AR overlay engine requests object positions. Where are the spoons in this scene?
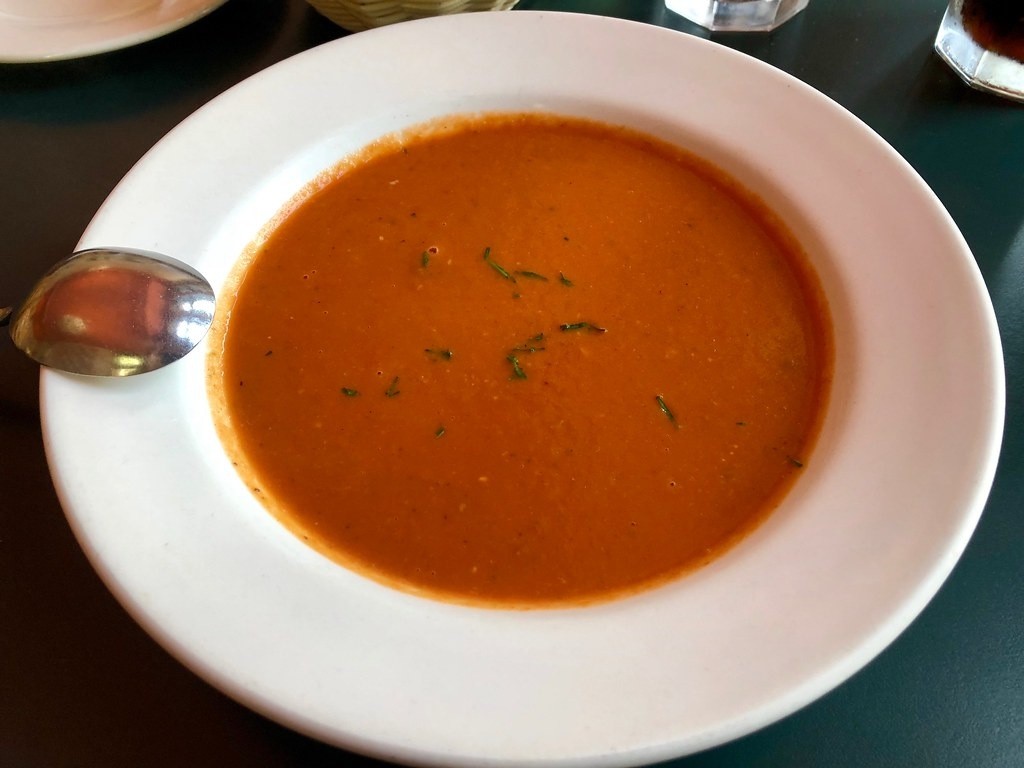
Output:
[0,245,217,378]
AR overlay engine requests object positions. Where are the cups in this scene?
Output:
[932,0,1024,99]
[663,0,809,32]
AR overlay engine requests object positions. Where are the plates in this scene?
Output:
[38,10,1006,768]
[0,0,228,64]
